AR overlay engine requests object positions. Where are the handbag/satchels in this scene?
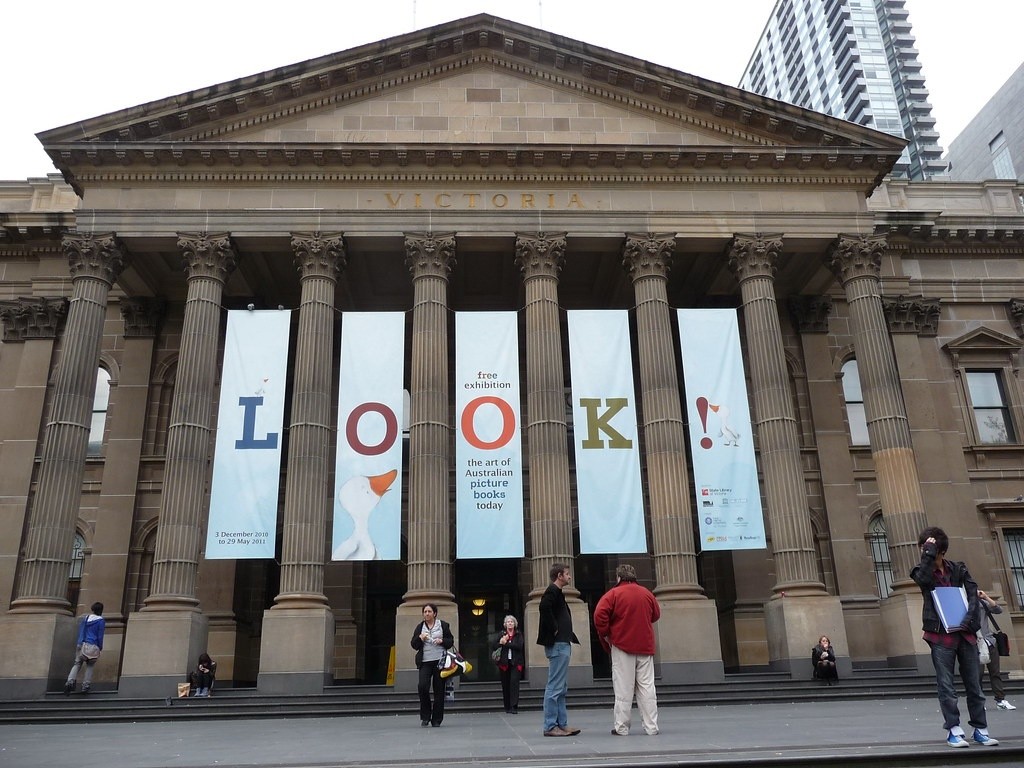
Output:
[438,644,473,678]
[994,631,1010,656]
[977,635,994,664]
[491,630,504,661]
[80,642,100,664]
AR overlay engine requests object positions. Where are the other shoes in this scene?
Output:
[432,722,440,727]
[420,720,430,726]
[824,679,835,686]
[63,683,73,697]
[611,729,619,735]
[543,726,581,736]
[506,708,518,714]
[194,687,208,697]
[81,684,92,692]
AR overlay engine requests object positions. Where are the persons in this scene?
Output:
[536,563,581,736]
[594,564,660,735]
[492,615,523,714]
[63,602,105,697]
[910,526,999,748]
[193,653,217,696]
[976,590,1016,711]
[410,603,454,728]
[812,636,839,686]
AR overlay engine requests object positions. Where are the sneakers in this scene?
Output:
[996,700,1016,710]
[946,726,969,748]
[970,727,999,746]
